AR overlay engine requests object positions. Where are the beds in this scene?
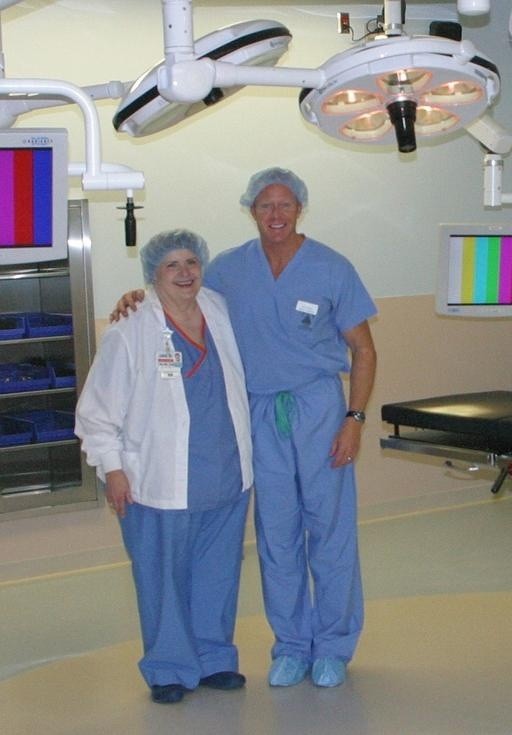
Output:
[380,388,511,494]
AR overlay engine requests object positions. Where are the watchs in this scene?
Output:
[346,410,367,421]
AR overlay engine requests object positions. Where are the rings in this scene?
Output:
[344,455,353,462]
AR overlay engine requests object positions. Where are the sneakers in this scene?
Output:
[150,685,184,705]
[198,672,246,691]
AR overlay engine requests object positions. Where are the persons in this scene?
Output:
[74,229,255,704]
[106,166,379,690]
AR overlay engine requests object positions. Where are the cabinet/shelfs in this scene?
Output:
[0,200,96,517]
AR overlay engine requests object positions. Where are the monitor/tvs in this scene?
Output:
[435,223,512,318]
[0,128,69,281]
